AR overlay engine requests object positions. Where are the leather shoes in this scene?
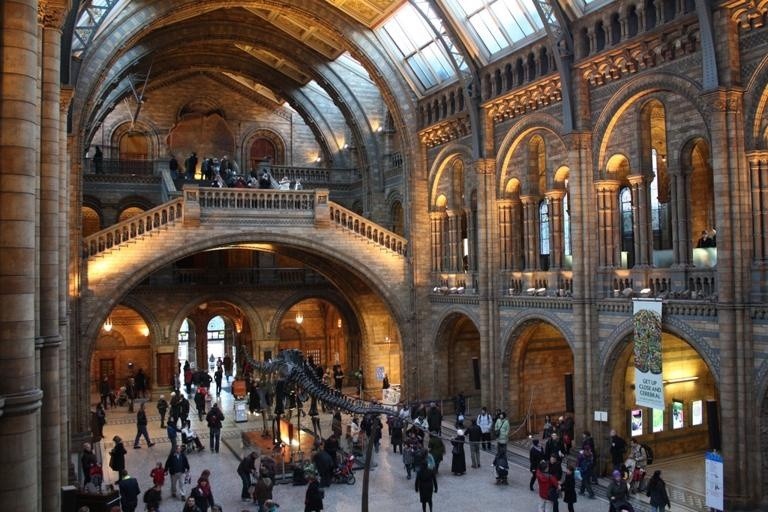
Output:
[148,443,155,447]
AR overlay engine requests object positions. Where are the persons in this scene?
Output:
[708,226,715,247]
[386,391,510,485]
[415,461,439,511]
[157,351,391,511]
[168,152,272,189]
[695,228,712,249]
[92,145,105,176]
[73,367,167,511]
[528,414,671,511]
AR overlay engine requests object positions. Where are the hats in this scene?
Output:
[160,394,164,399]
[170,391,176,396]
[613,470,620,477]
[610,429,616,436]
[133,446,141,449]
[456,429,464,436]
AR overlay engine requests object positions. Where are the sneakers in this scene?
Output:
[172,493,176,496]
[181,495,185,501]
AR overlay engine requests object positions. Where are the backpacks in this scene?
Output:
[640,442,653,465]
[478,413,490,425]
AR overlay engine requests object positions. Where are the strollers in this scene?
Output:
[333,454,356,485]
[623,456,638,496]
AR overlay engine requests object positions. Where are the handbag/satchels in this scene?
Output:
[494,429,500,436]
[547,485,558,499]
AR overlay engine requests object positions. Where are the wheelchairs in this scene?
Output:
[179,427,203,453]
